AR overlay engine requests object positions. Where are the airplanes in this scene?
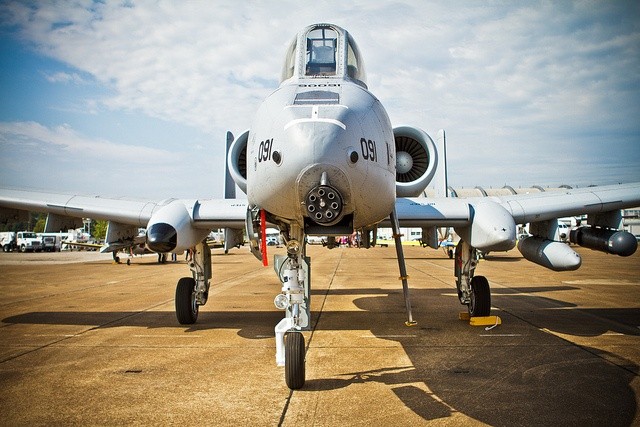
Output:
[370,227,519,258]
[0,25,640,389]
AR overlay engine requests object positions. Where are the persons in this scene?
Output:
[306,64,312,76]
[347,65,368,89]
[171,253,178,262]
[184,249,195,261]
[158,252,167,263]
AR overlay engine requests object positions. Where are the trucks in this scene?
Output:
[0,230,44,252]
